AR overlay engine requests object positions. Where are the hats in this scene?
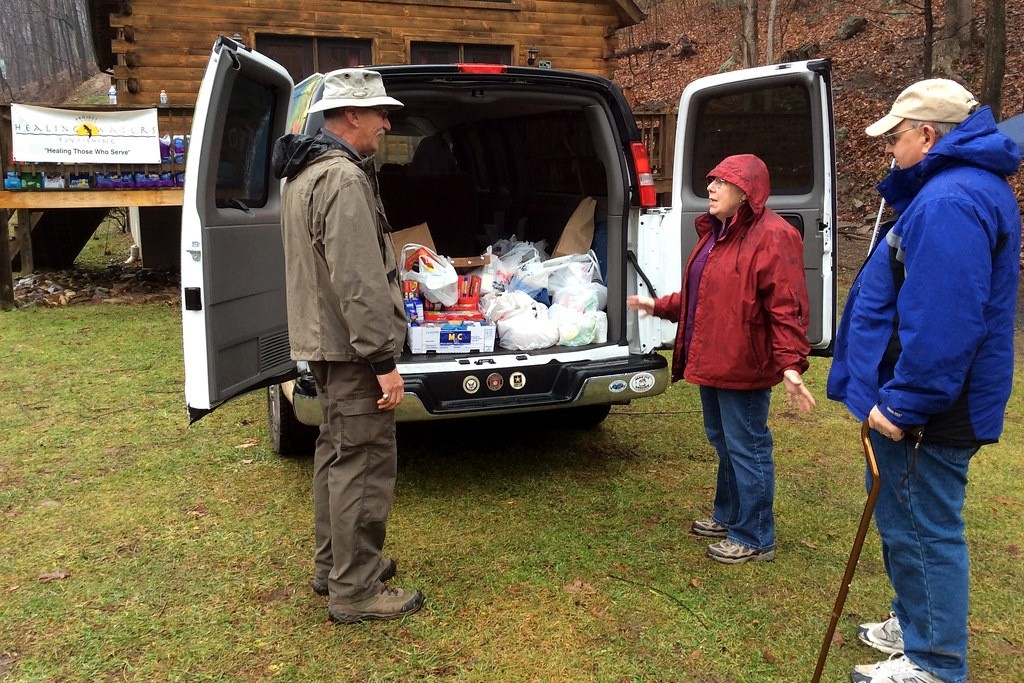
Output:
[865,78,979,138]
[305,68,405,114]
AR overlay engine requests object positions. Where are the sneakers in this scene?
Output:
[851,654,947,683]
[329,584,424,625]
[312,557,397,597]
[691,518,730,538]
[857,611,906,657]
[706,538,776,564]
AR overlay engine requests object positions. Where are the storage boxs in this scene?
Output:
[406,304,499,355]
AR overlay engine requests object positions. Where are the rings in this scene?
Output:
[380,393,393,401]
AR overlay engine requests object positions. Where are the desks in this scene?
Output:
[0,188,185,308]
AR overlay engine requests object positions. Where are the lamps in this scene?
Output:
[527,43,538,65]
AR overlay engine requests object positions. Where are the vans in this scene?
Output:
[179,33,839,457]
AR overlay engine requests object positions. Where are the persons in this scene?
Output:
[273,67,429,626]
[630,154,817,566]
[828,76,1024,683]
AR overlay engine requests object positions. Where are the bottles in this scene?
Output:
[160,90,167,104]
[108,86,117,104]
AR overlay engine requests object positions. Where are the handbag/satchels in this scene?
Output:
[479,242,608,351]
[550,196,597,258]
[399,244,459,308]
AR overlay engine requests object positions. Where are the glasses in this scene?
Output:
[882,125,915,146]
[706,176,728,191]
[363,107,388,118]
